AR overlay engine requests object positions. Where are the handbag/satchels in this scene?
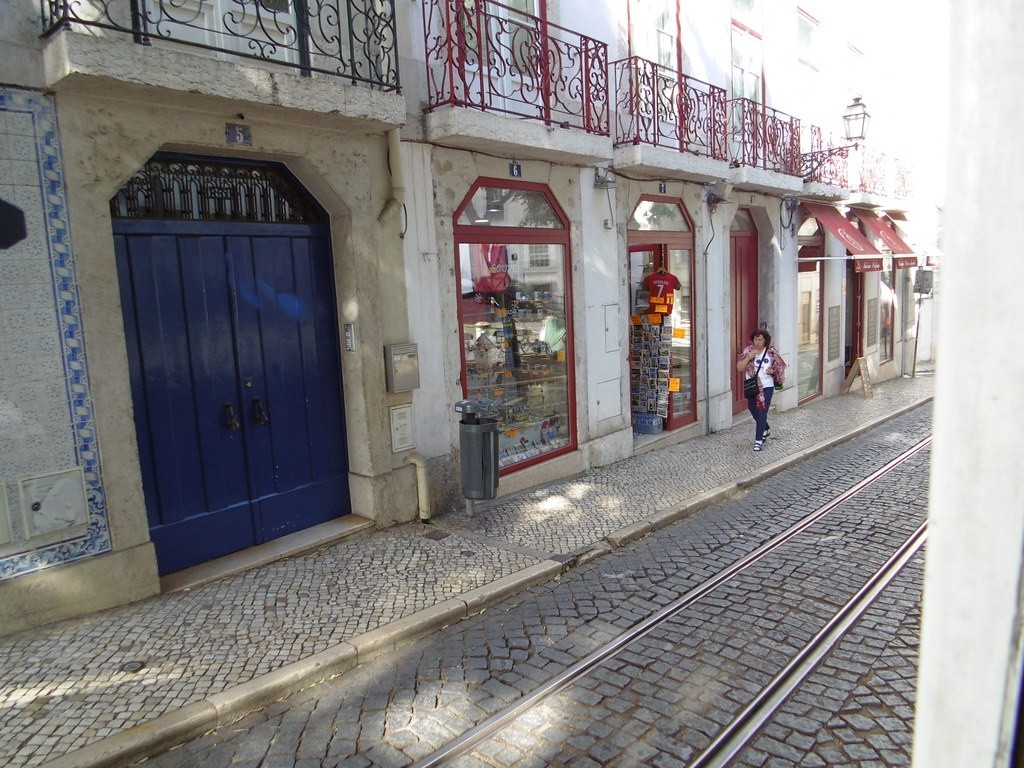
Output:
[744,377,760,398]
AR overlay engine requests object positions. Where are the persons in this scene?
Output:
[737,328,786,451]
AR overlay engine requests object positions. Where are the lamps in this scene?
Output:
[707,181,734,209]
[795,97,871,177]
[593,167,617,191]
[782,195,799,211]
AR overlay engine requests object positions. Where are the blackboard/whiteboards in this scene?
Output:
[842,357,872,394]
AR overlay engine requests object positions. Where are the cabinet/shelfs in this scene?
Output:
[468,375,568,464]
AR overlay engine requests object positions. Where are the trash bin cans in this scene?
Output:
[454,398,499,501]
[474,321,491,339]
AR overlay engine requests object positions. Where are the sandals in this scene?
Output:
[761,429,770,443]
[754,442,762,451]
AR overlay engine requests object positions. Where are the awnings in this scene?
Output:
[889,208,942,267]
[850,201,918,271]
[798,199,885,275]
